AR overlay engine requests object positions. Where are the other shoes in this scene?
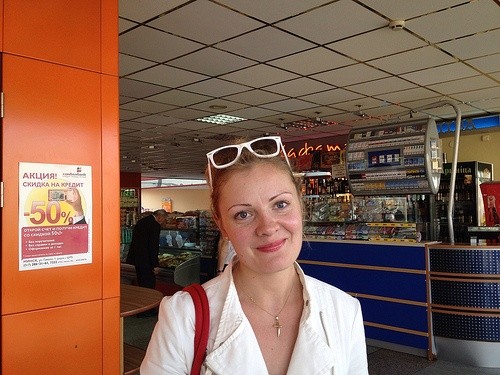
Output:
[137,309,156,318]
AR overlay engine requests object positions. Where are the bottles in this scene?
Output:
[435,182,472,202]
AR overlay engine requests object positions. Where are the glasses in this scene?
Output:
[206,136,294,190]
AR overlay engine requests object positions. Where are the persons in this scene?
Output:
[124,209,169,292]
[140,135,368,375]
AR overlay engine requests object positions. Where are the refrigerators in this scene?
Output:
[437,161,494,243]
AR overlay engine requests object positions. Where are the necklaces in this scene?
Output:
[232,275,298,336]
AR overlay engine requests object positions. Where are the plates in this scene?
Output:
[478,243,487,246]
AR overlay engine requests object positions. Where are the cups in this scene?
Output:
[479,239,486,243]
[470,236,477,246]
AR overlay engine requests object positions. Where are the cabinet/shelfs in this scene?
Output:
[120,282,163,375]
[118,174,427,290]
